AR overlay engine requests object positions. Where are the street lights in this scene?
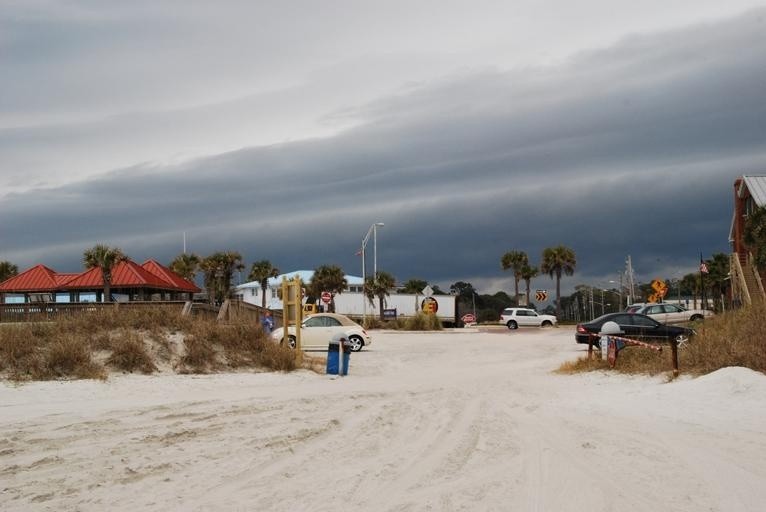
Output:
[373,222,386,281]
[581,279,638,315]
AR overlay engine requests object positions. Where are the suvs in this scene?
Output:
[498,306,560,330]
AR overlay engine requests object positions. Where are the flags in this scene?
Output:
[700,260,711,275]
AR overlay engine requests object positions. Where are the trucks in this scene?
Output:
[301,292,460,330]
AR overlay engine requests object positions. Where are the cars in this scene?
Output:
[632,303,715,326]
[267,314,372,354]
[574,313,698,350]
[622,301,644,314]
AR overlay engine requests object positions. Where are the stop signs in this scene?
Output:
[319,291,333,304]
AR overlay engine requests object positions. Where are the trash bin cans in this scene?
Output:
[598,321,625,360]
[326,333,351,376]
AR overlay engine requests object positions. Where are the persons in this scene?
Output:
[260,309,275,333]
